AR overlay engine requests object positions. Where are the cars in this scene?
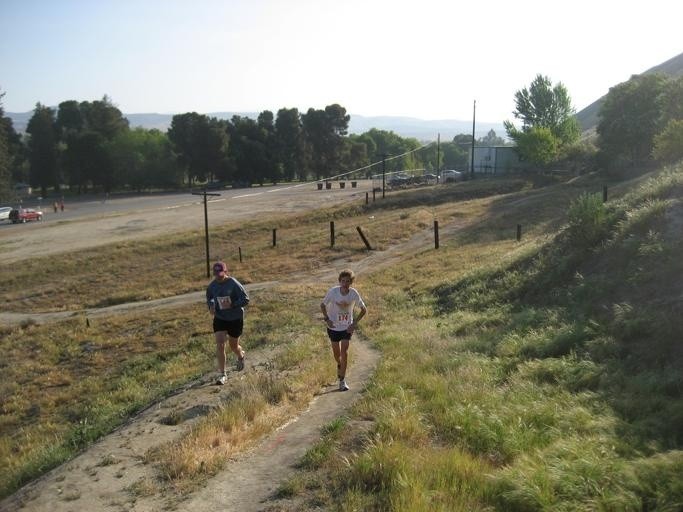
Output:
[388,169,462,186]
[0,205,43,225]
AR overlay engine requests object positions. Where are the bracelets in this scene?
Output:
[324,316,329,321]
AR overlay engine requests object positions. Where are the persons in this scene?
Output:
[54,200,58,212]
[59,198,64,212]
[207,263,249,385]
[321,271,366,391]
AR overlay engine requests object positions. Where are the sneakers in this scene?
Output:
[337,362,342,378]
[236,350,246,371]
[339,378,349,391]
[216,370,228,385]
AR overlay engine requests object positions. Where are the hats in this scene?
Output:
[213,262,227,276]
